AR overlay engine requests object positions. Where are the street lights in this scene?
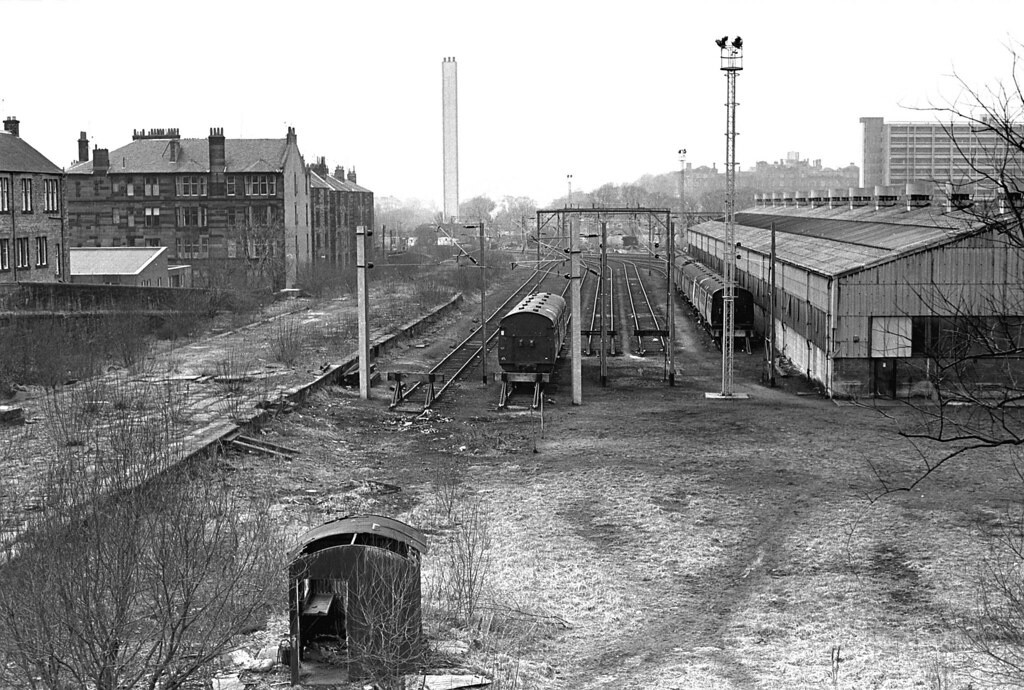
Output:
[678,146,687,241]
[716,35,742,398]
[566,171,575,212]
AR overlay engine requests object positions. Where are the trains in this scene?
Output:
[667,248,756,344]
[491,291,569,385]
